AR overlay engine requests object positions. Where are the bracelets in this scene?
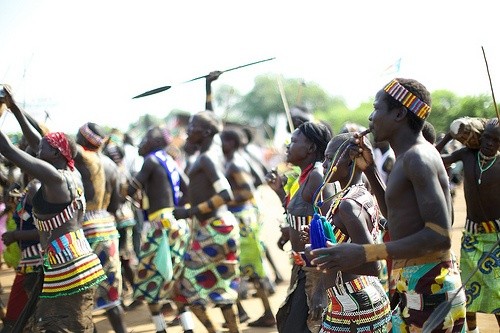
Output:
[227,200,238,206]
[187,209,193,218]
[281,194,289,208]
[383,220,388,232]
[362,242,388,264]
[206,94,212,103]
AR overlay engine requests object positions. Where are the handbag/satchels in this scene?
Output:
[275,268,312,333]
[3,272,29,321]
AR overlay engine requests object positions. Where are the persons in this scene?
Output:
[309,77,466,333]
[422,114,500,333]
[0,70,396,333]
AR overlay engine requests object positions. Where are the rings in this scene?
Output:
[268,178,272,182]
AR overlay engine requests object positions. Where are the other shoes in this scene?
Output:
[247,315,276,327]
[166,316,180,326]
[240,313,249,323]
[252,286,276,297]
[123,299,143,311]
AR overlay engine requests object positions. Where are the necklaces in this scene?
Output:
[343,182,365,192]
[477,150,499,185]
